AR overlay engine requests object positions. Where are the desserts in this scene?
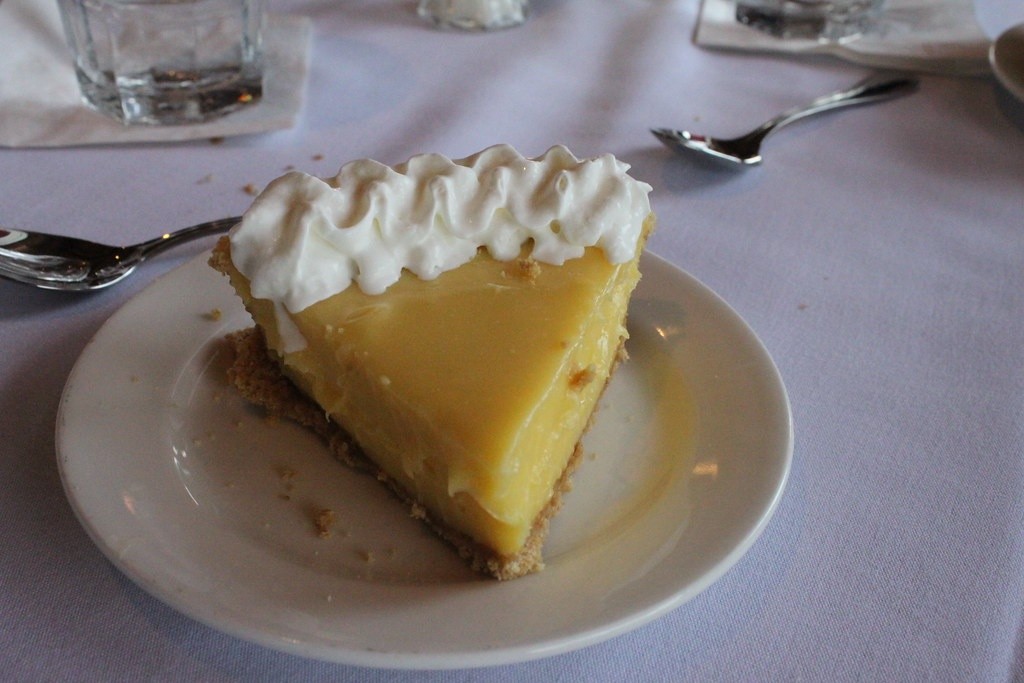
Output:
[207,144,656,583]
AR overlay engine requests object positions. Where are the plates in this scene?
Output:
[54,247,795,671]
[987,21,1024,105]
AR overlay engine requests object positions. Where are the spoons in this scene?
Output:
[0,216,243,290]
[650,69,921,170]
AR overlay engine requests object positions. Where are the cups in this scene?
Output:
[734,0,887,45]
[55,0,265,126]
